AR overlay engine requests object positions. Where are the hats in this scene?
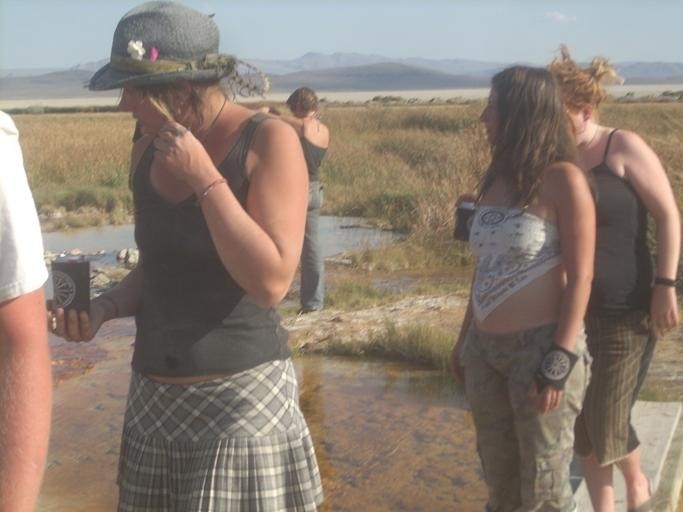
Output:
[86,0,238,91]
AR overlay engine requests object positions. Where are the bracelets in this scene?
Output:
[195,179,227,207]
[655,277,680,288]
[102,295,120,317]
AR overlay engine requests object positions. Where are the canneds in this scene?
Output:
[50,251,89,318]
[454,202,474,241]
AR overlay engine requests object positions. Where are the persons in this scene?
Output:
[458,46,682,512]
[259,86,330,313]
[1,111,52,512]
[450,64,596,512]
[45,1,325,510]
